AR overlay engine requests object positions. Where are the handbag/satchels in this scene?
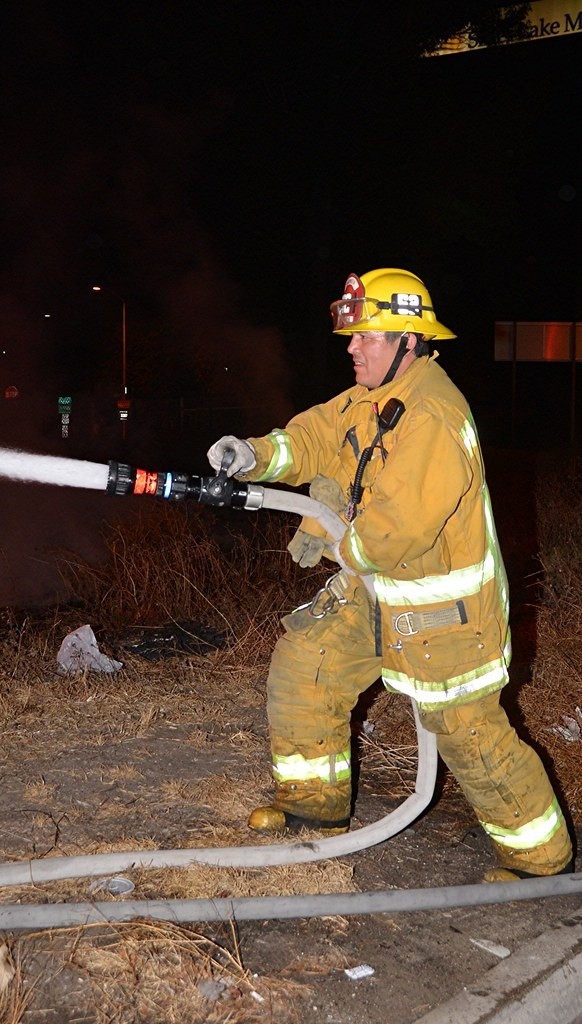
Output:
[57,624,123,677]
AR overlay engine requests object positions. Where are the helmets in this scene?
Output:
[330,267,457,342]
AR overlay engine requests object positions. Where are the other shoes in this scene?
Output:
[482,865,574,884]
[247,805,350,835]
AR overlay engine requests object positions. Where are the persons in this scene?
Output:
[205,267,576,884]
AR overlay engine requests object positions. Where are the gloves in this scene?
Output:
[206,435,257,478]
[287,473,346,568]
